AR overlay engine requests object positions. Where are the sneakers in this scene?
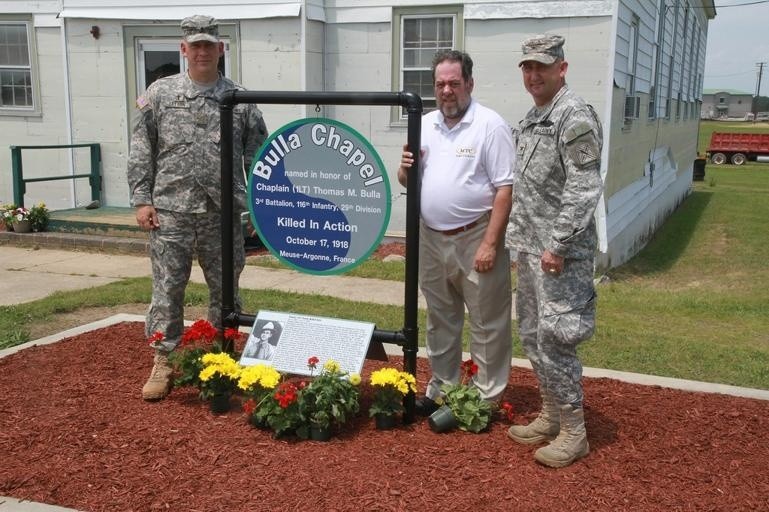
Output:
[415,395,438,415]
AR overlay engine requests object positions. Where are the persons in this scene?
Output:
[506,32,604,469]
[244,321,274,360]
[127,14,269,400]
[396,50,515,417]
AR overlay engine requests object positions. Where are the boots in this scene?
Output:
[508,398,560,444]
[143,350,175,400]
[534,399,589,468]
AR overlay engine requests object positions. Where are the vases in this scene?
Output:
[7,222,44,232]
[3,203,50,229]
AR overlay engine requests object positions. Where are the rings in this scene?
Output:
[550,269,555,272]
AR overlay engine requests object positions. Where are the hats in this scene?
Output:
[260,322,278,334]
[519,33,564,67]
[181,14,219,43]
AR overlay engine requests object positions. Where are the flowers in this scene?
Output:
[147,319,250,353]
[199,352,281,391]
[370,366,418,396]
[275,382,298,410]
[496,400,513,422]
[243,398,259,417]
[300,355,362,393]
[458,357,480,386]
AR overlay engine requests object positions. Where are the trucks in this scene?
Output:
[706,132,769,166]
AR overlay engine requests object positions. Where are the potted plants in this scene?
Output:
[427,403,456,434]
[374,409,398,432]
[206,390,230,418]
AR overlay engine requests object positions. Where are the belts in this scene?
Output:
[427,221,476,236]
